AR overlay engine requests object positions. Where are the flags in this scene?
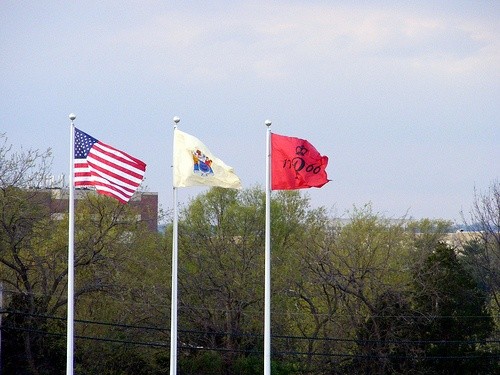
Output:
[269,132,331,190]
[173,127,243,190]
[73,126,147,206]
